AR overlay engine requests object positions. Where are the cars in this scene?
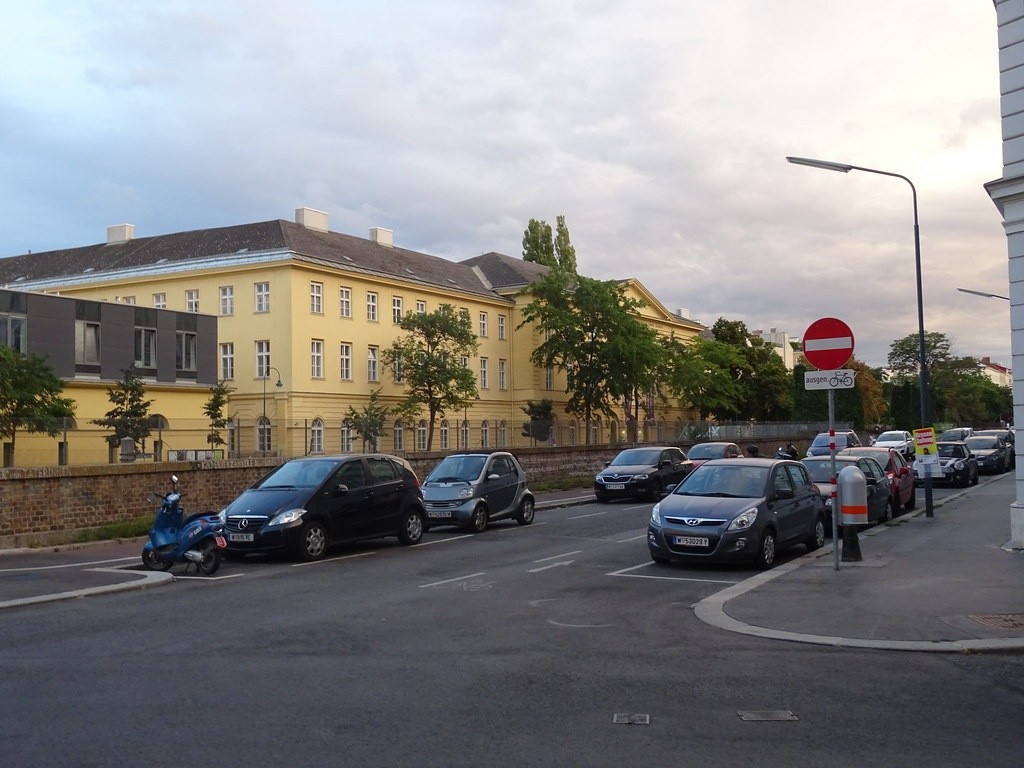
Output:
[593,446,697,504]
[835,445,917,518]
[419,450,537,534]
[940,426,1015,468]
[871,429,915,462]
[646,456,828,571]
[788,455,897,540]
[962,434,1010,474]
[687,442,745,468]
[911,440,978,489]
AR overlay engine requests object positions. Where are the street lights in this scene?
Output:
[262,366,285,456]
[786,154,934,518]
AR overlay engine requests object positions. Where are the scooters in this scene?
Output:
[140,475,229,576]
[747,441,800,462]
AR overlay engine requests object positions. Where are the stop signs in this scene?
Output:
[800,317,855,371]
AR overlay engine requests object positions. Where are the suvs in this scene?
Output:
[215,453,427,562]
[805,429,863,457]
